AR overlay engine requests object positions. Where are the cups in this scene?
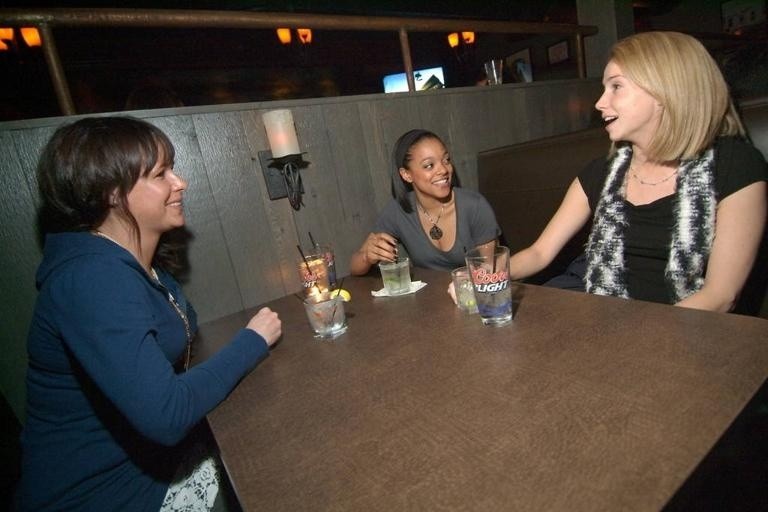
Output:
[485,59,502,85]
[298,245,350,341]
[452,243,512,329]
[379,257,414,295]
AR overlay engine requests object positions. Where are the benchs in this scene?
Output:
[476,104,766,314]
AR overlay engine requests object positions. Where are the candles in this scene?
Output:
[260,108,302,160]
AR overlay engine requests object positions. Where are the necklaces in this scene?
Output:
[626,162,679,185]
[419,204,446,240]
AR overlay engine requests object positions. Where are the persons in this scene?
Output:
[20,115,285,512]
[445,30,767,314]
[349,130,516,273]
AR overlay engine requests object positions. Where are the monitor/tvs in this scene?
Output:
[379,61,449,93]
